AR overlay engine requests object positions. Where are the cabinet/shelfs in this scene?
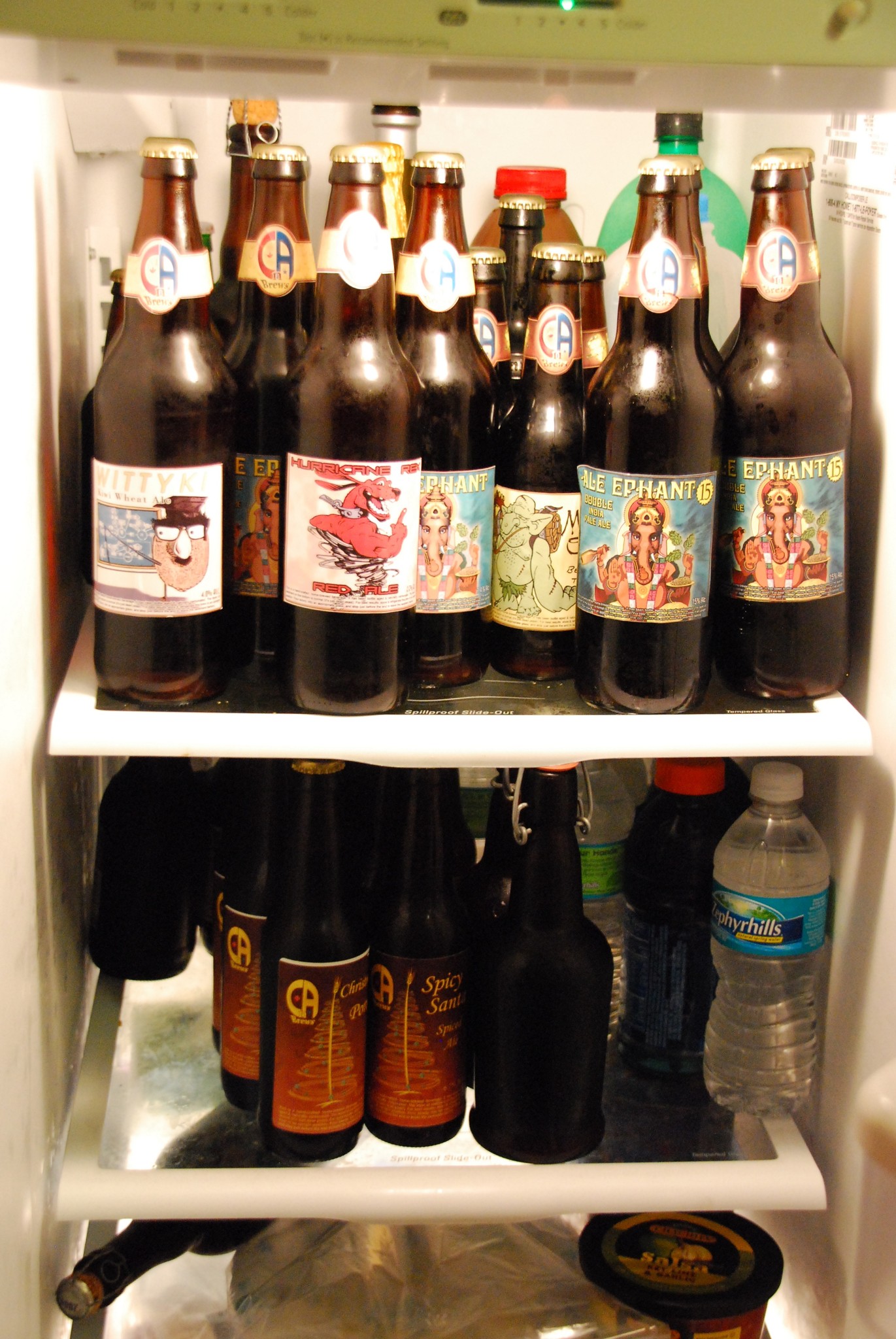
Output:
[36,579,877,1213]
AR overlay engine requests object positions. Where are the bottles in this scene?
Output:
[80,138,226,707]
[371,103,421,224]
[208,98,282,339]
[574,759,653,1042]
[483,242,609,682]
[280,142,428,716]
[717,148,853,701]
[198,757,257,1113]
[614,757,732,1087]
[396,152,497,692]
[221,144,316,662]
[256,757,365,1163]
[576,155,726,715]
[469,166,584,369]
[85,757,198,980]
[702,762,832,1118]
[55,1100,280,1322]
[469,245,512,434]
[364,764,475,1148]
[469,765,614,1164]
[598,113,750,354]
[485,195,546,434]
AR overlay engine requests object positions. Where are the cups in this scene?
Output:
[578,1212,785,1339]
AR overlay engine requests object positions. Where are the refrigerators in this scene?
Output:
[0,0,896,1338]
